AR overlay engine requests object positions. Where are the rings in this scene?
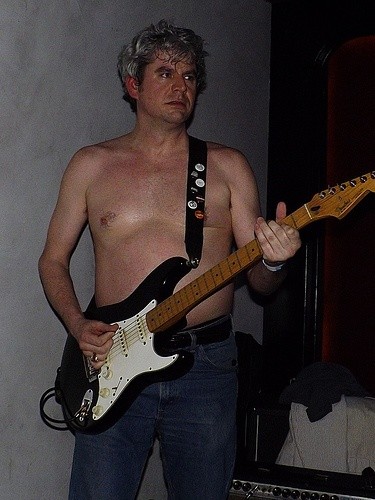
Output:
[91,352,98,362]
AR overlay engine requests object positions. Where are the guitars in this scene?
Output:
[55,171,375,435]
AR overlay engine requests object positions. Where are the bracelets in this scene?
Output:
[262,260,286,272]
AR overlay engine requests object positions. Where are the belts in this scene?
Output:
[170,316,231,348]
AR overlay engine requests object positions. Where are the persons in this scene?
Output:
[38,18,301,500]
